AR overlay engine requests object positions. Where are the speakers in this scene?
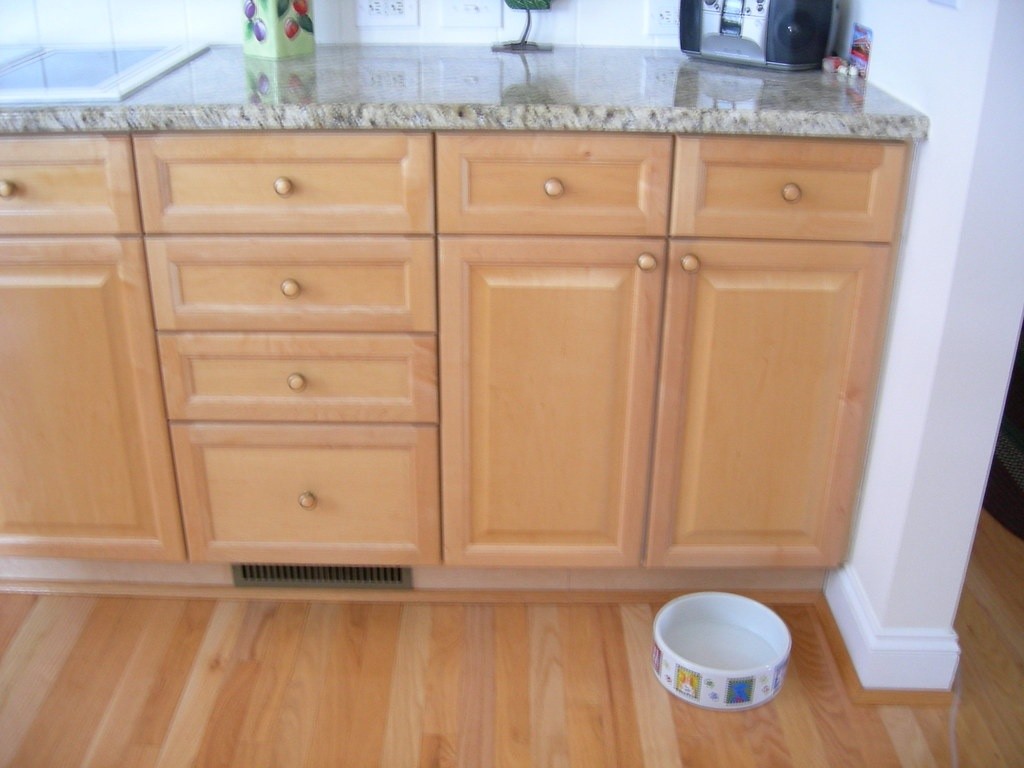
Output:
[767,0,840,70]
[679,0,702,59]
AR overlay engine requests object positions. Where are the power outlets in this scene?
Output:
[644,0,680,35]
[354,1,422,26]
[440,1,503,30]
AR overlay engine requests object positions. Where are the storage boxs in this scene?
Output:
[242,55,316,104]
[241,0,316,61]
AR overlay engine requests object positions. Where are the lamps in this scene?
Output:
[491,0,552,53]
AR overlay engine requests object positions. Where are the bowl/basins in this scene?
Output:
[651,591,791,708]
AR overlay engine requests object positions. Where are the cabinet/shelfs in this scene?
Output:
[1,133,184,562]
[437,130,908,570]
[134,129,442,572]
